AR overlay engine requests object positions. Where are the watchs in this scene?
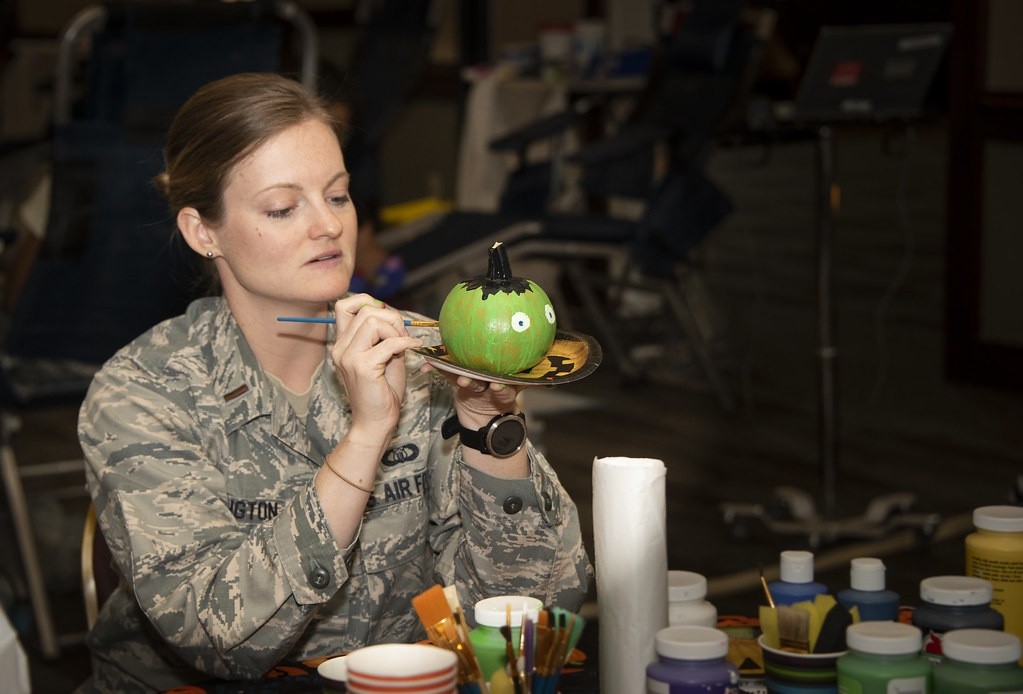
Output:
[442,413,527,458]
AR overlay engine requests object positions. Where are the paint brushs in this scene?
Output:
[758,566,776,609]
[277,316,440,328]
[412,582,581,694]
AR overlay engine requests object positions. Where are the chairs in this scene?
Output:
[0,0,743,660]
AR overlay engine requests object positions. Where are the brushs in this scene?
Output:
[776,606,810,653]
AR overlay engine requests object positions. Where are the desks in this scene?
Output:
[456,78,722,360]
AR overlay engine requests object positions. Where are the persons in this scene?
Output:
[77,74,593,694]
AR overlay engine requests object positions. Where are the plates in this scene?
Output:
[412,327,603,386]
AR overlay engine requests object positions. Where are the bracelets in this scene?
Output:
[325,454,375,492]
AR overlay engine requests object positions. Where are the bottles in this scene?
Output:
[913,575,1004,638]
[931,627,1023,694]
[467,594,545,694]
[964,505,1023,668]
[767,550,828,607]
[666,570,717,628]
[836,620,933,694]
[835,556,901,634]
[644,625,740,694]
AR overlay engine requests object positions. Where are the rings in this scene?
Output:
[472,383,489,394]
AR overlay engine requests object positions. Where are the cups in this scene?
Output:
[344,641,460,694]
[759,634,848,694]
[316,656,347,694]
